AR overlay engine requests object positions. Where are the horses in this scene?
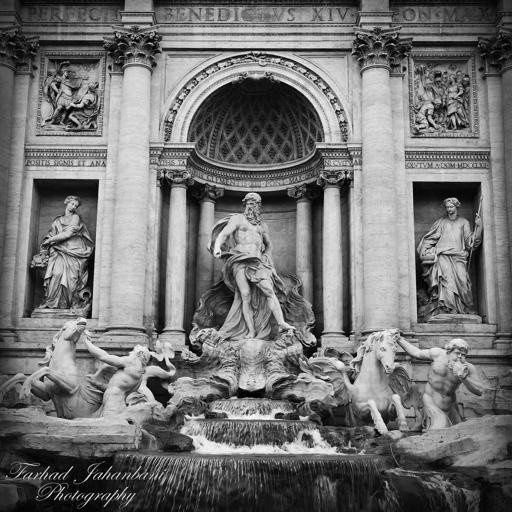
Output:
[0,316,102,420]
[342,328,410,436]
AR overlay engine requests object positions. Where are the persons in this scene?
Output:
[391,327,484,433]
[417,197,482,322]
[82,328,176,420]
[188,192,317,346]
[30,195,95,310]
[40,60,103,130]
[411,63,471,134]
